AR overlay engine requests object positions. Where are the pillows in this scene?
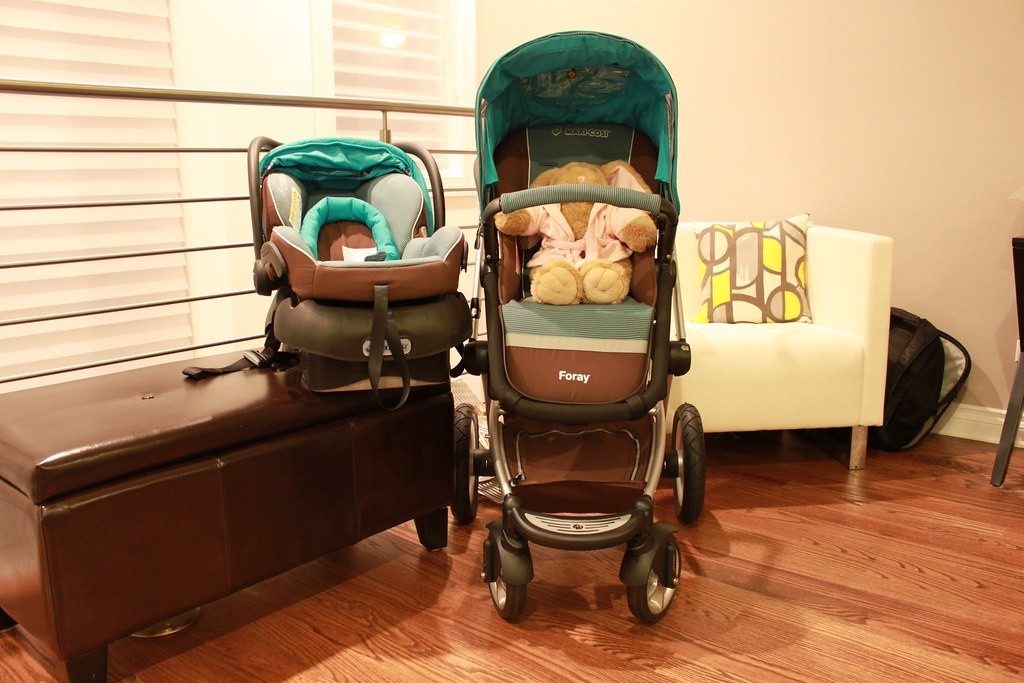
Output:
[692,212,812,325]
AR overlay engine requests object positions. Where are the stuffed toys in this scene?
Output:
[491,160,659,304]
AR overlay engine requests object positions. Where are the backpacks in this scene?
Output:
[789,307,973,454]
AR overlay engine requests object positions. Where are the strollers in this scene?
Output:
[450,29,707,625]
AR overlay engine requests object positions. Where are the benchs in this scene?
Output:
[0,348,454,683]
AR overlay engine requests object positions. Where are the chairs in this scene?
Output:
[247,137,473,363]
[651,222,893,471]
